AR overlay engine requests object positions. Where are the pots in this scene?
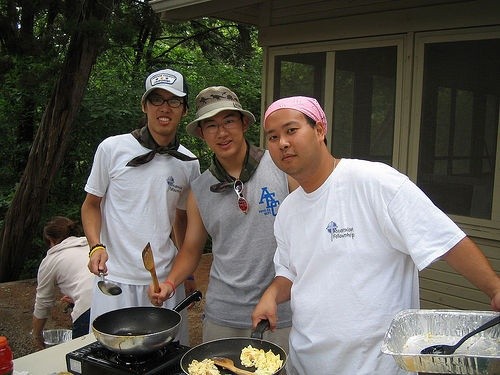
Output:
[179,317,287,375]
[92,290,202,355]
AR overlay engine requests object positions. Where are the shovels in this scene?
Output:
[211,357,255,375]
[142,242,160,293]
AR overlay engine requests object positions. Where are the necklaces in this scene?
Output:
[334,158,335,169]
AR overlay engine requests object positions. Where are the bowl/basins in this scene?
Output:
[42,329,72,348]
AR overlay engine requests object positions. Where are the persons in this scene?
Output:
[32,216,97,349]
[251,96,500,375]
[79,69,201,347]
[147,86,301,355]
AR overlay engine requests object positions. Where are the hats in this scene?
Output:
[141,68,189,117]
[186,85,256,138]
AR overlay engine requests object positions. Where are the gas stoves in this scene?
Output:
[65,338,197,375]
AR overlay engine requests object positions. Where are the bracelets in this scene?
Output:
[187,276,194,280]
[89,244,106,257]
[165,282,175,297]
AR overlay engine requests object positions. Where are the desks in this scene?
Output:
[13,333,96,375]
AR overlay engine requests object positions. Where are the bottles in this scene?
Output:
[0,335,14,375]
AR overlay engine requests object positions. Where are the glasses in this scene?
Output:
[147,96,185,108]
[234,178,249,214]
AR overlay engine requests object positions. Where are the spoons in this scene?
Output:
[420,315,500,355]
